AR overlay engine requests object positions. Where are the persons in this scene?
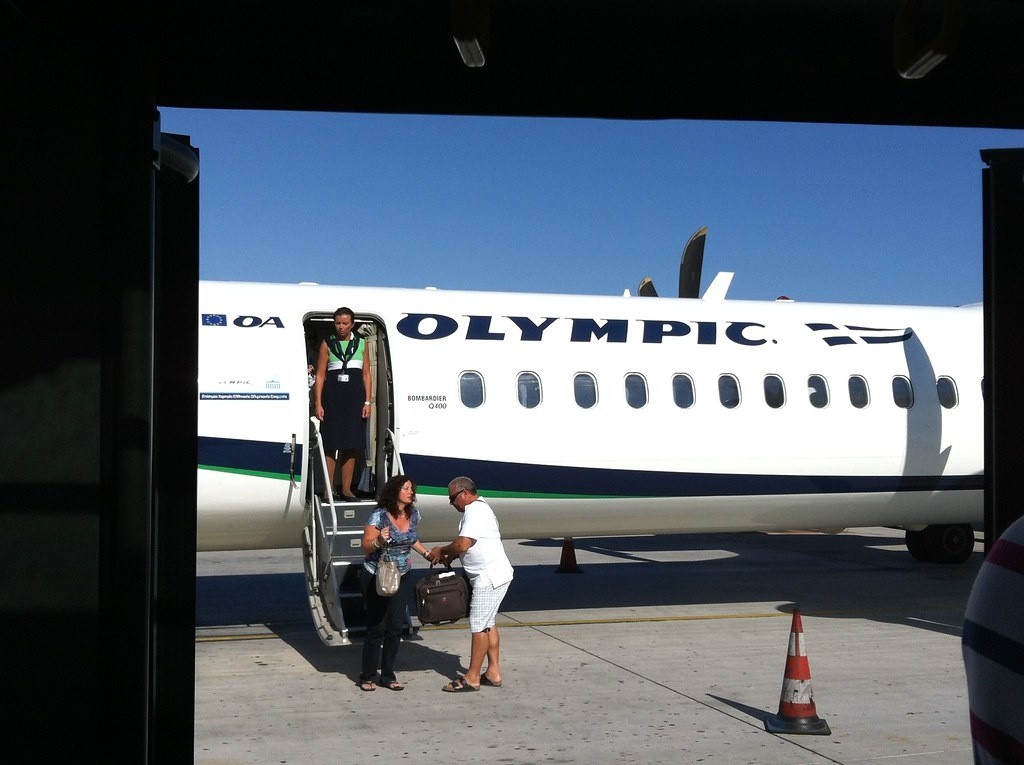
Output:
[360,475,446,691]
[314,306,372,504]
[426,477,515,692]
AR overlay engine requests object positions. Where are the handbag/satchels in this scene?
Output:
[415,561,470,626]
[376,548,401,595]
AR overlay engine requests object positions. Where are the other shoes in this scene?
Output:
[320,496,335,504]
[340,493,360,502]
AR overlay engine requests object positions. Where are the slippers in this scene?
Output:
[442,677,480,691]
[479,673,502,687]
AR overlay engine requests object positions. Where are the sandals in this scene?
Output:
[359,676,376,691]
[381,681,404,690]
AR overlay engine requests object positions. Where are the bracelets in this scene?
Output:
[423,551,430,558]
[365,401,372,406]
[371,539,382,550]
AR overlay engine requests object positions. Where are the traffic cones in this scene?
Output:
[765,608,831,736]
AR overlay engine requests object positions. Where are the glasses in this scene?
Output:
[449,489,464,502]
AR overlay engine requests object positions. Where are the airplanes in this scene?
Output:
[196,224,986,565]
